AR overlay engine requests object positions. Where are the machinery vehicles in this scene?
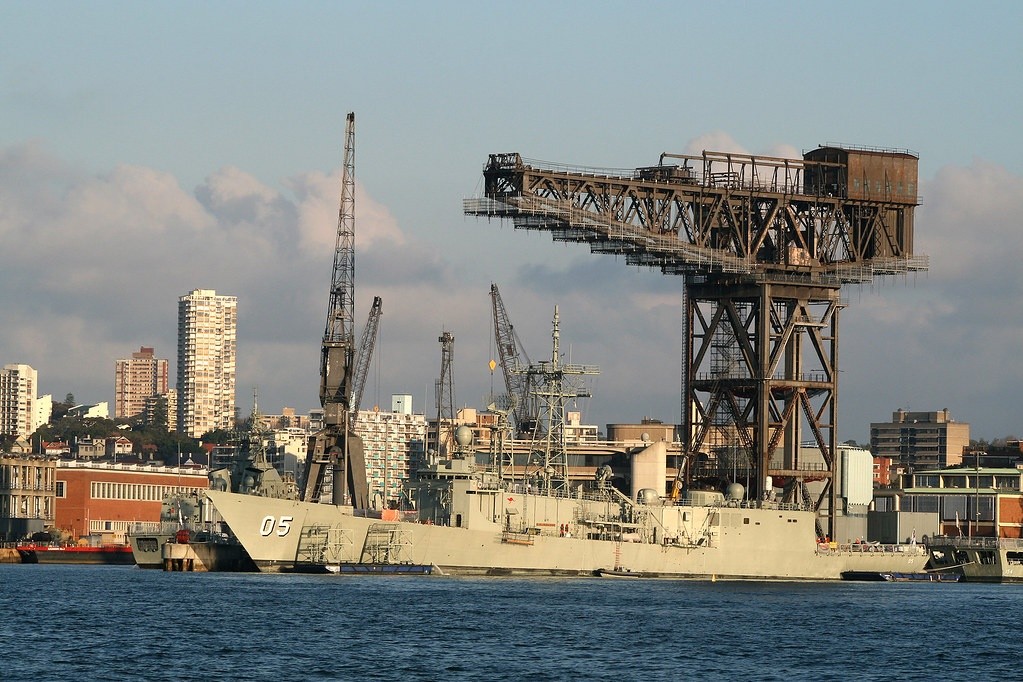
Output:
[433,322,456,470]
[485,283,552,444]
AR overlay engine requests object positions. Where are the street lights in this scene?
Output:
[969,449,986,543]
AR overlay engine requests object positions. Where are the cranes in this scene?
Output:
[301,110,385,510]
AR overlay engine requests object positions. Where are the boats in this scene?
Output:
[201,489,930,583]
[924,536,1023,583]
[15,544,134,568]
